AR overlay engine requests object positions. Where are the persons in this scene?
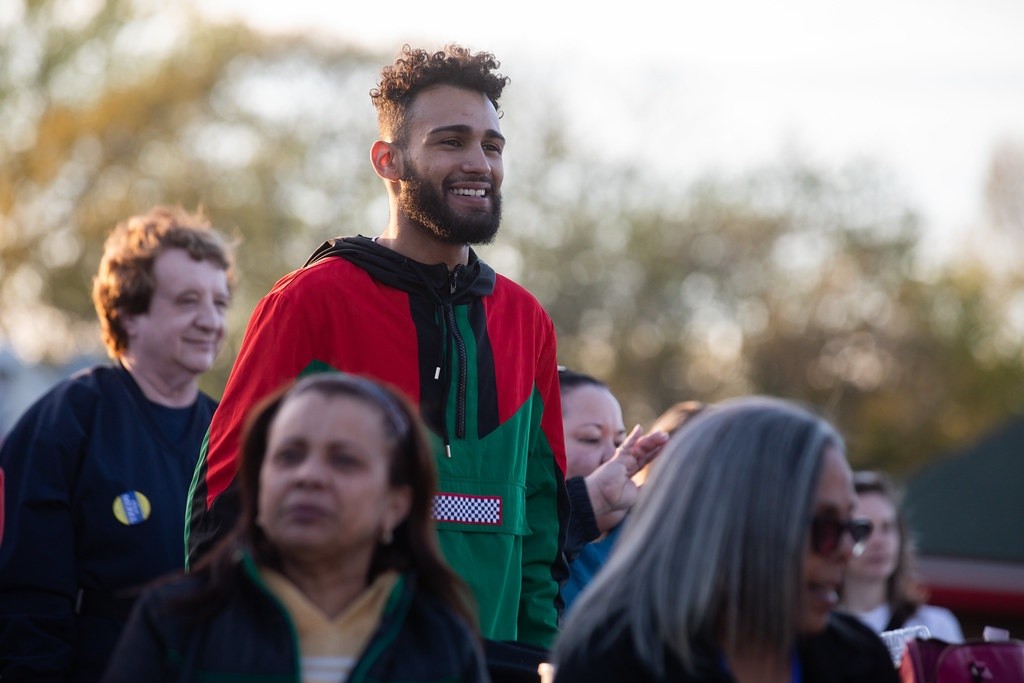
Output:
[829,473,967,683]
[551,398,902,683]
[104,373,491,683]
[0,210,234,683]
[557,366,705,628]
[183,39,571,683]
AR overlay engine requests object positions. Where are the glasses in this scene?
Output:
[812,512,875,556]
